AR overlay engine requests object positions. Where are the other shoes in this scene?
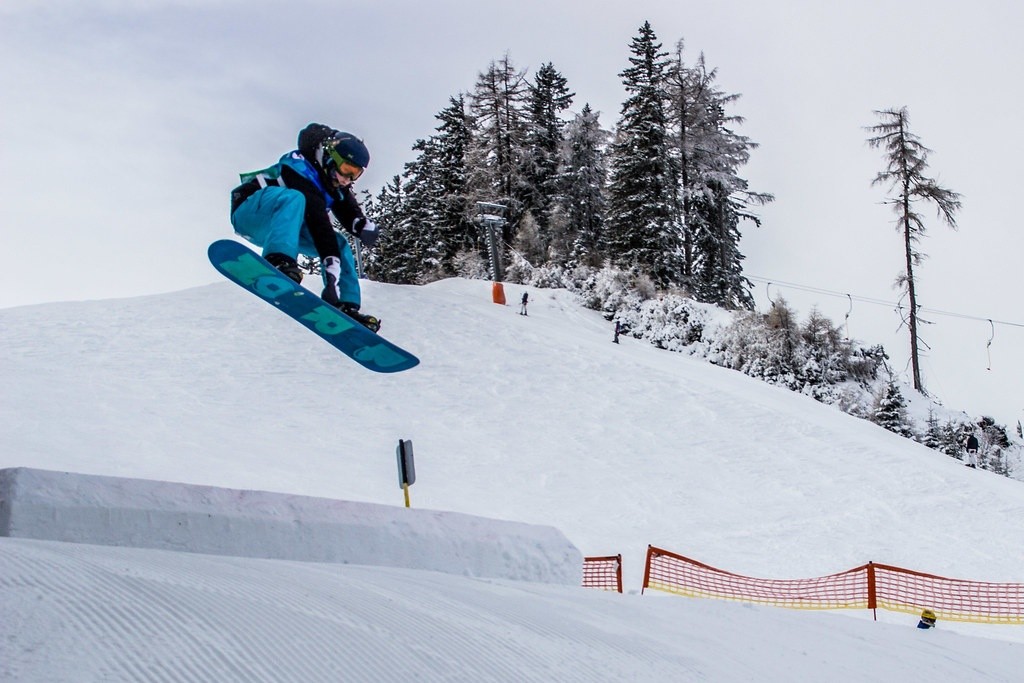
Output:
[270,259,303,284]
[336,303,379,333]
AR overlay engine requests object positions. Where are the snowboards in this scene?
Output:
[208,237,420,375]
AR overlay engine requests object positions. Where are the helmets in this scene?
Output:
[325,131,370,174]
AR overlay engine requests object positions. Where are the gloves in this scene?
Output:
[352,217,378,245]
[322,256,341,304]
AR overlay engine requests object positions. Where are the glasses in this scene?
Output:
[326,141,363,181]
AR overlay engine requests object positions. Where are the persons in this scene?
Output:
[965,432,978,469]
[230,122,382,335]
[612,321,620,344]
[520,291,528,316]
